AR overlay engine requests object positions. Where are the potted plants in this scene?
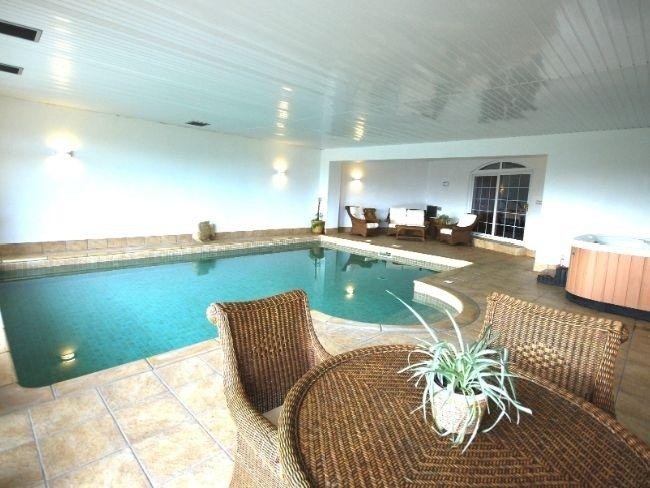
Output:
[379,286,533,457]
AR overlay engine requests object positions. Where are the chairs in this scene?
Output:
[345,205,479,246]
[204,289,334,487]
[472,292,631,422]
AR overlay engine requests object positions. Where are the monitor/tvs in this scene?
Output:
[426,205,438,217]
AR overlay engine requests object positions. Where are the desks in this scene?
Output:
[278,343,650,488]
